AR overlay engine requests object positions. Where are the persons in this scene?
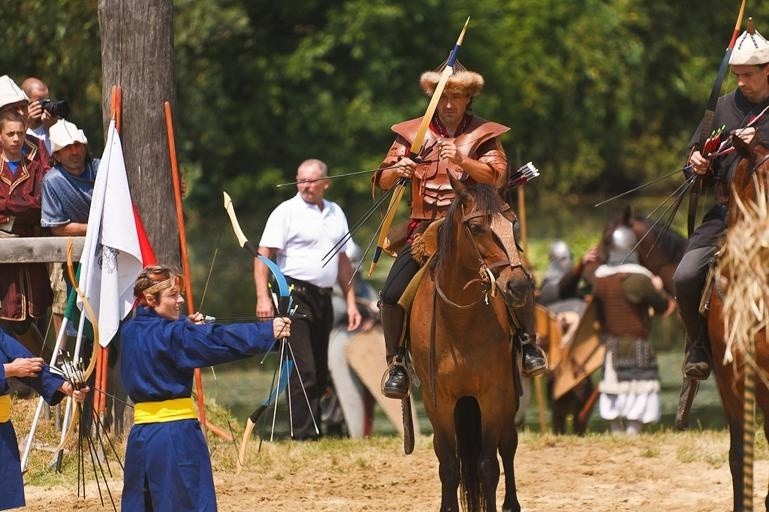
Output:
[533,240,598,436]
[381,51,548,395]
[117,264,291,512]
[0,329,91,512]
[673,32,769,383]
[41,120,118,372]
[0,110,49,359]
[593,227,673,436]
[20,77,57,158]
[252,158,362,439]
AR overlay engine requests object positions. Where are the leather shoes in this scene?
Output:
[520,333,548,377]
[383,366,411,398]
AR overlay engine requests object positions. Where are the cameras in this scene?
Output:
[39,97,71,119]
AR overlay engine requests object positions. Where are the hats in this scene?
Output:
[47,117,89,157]
[726,15,769,66]
[418,49,486,97]
[610,225,638,251]
[547,240,575,263]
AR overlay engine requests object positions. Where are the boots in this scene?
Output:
[677,308,714,382]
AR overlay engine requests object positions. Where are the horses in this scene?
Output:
[578,202,701,433]
[404,165,537,512]
[701,130,768,512]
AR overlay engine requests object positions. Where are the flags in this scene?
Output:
[76,119,144,347]
